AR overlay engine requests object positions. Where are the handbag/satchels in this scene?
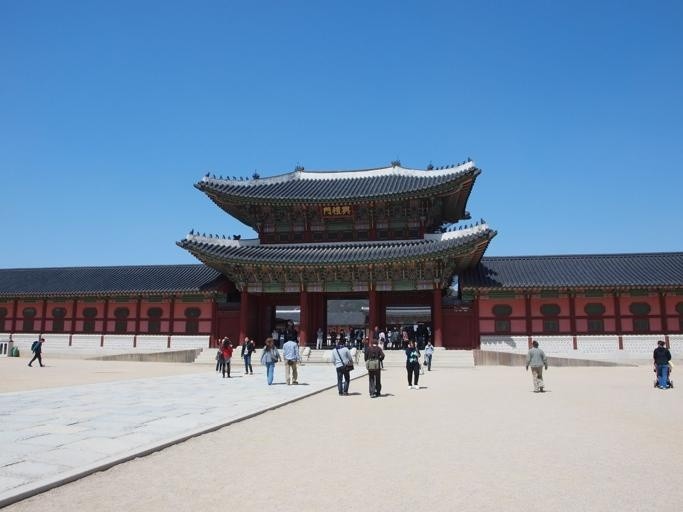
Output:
[345,363,354,371]
[366,359,380,371]
[271,354,278,362]
[424,354,427,365]
[381,337,384,342]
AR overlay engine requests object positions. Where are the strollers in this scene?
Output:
[653,361,673,388]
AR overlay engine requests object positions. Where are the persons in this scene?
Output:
[211,317,435,390]
[27,339,44,367]
[524,341,548,392]
[331,341,352,395]
[363,340,384,398]
[652,341,671,390]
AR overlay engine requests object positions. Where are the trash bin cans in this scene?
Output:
[0,341,13,357]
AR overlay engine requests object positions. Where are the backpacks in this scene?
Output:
[31,341,38,350]
